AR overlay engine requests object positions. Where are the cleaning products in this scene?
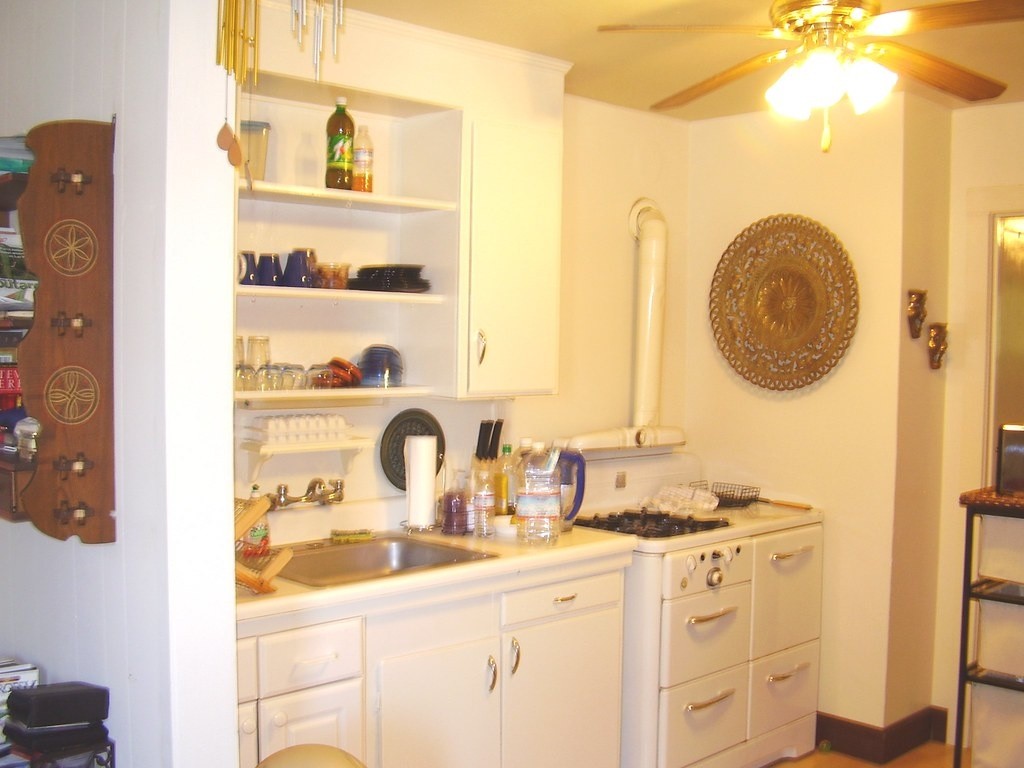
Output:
[243,484,271,558]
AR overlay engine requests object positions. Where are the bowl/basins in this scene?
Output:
[356,343,404,387]
[312,262,350,289]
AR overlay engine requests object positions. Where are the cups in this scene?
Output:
[235,335,333,392]
[237,248,314,288]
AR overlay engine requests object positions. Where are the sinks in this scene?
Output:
[243,537,500,589]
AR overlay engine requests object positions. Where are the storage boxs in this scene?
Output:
[2,680,109,753]
[0,664,39,759]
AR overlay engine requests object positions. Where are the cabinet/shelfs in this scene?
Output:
[622,523,824,768]
[233,1,574,402]
[0,120,117,544]
[953,485,1023,768]
[379,570,624,768]
[237,614,368,768]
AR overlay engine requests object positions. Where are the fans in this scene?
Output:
[596,0,1024,115]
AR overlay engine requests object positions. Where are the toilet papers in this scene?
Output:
[403,435,438,528]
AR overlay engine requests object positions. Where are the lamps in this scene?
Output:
[764,45,899,151]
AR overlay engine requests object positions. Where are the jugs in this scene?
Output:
[553,438,588,532]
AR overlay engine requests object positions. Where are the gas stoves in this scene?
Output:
[573,507,745,554]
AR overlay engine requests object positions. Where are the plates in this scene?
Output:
[346,263,431,293]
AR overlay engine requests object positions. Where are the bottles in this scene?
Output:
[442,437,561,548]
[240,119,270,182]
[243,484,271,557]
[325,95,355,191]
[351,125,375,193]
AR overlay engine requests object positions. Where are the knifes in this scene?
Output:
[476,417,504,461]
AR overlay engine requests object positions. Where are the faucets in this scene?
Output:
[306,477,326,497]
